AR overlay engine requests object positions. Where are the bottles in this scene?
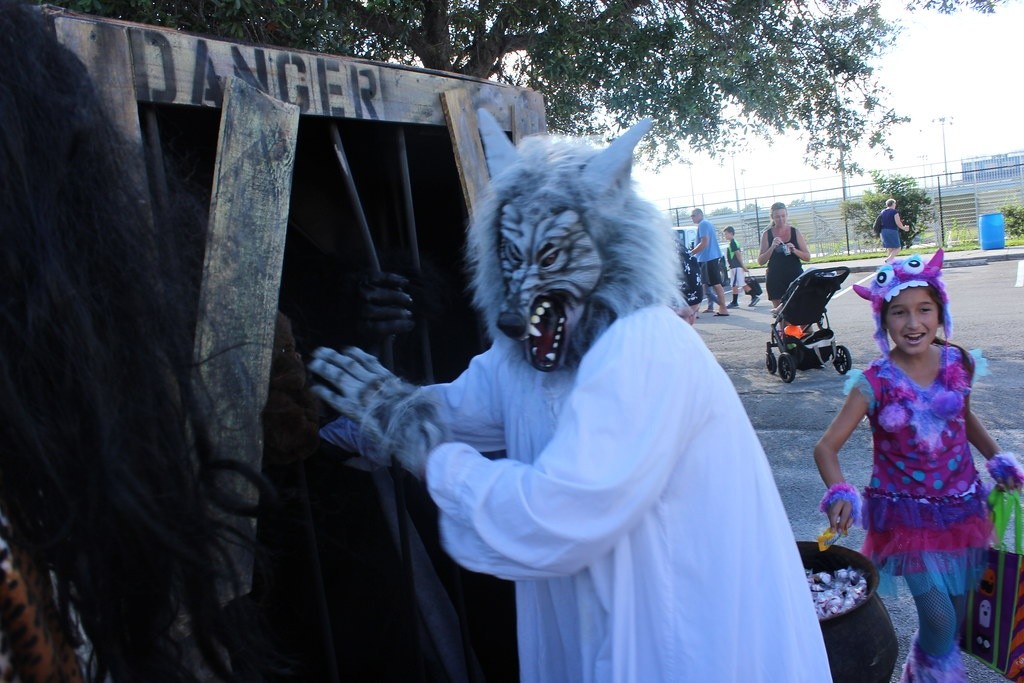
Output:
[795,537,898,683]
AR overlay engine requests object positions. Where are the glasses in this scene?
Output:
[691,215,696,217]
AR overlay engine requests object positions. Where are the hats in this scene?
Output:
[677,245,705,308]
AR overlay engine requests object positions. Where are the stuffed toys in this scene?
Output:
[307,105,834,683]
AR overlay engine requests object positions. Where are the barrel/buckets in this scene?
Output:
[978,211,1004,251]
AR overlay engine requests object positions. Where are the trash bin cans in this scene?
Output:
[977,212,1006,250]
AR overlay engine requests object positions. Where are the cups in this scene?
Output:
[781,244,791,255]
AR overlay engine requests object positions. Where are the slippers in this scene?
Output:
[702,310,714,313]
[713,312,729,316]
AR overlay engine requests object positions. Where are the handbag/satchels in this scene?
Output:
[873,213,884,235]
[744,271,762,296]
[958,485,1023,682]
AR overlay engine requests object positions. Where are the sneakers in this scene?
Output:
[726,303,740,309]
[748,297,760,307]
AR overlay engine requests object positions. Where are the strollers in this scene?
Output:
[764,265,853,384]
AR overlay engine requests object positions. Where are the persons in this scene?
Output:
[758,201,814,337]
[686,208,730,318]
[879,199,910,263]
[724,226,761,310]
[674,239,702,323]
[813,248,1024,683]
